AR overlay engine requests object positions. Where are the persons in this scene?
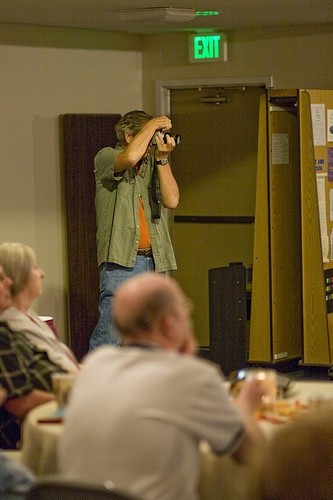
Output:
[1,242,82,376]
[52,270,278,499]
[87,110,181,354]
[0,261,79,452]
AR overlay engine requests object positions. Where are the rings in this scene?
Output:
[160,143,164,145]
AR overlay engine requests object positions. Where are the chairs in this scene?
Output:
[19,381,333,500]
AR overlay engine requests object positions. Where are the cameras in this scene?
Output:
[152,129,181,145]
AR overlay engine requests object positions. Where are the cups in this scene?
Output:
[51,373,74,410]
[309,393,333,411]
[246,369,279,408]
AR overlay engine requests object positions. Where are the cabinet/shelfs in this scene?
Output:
[209,265,253,375]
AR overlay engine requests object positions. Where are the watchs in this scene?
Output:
[155,158,169,166]
